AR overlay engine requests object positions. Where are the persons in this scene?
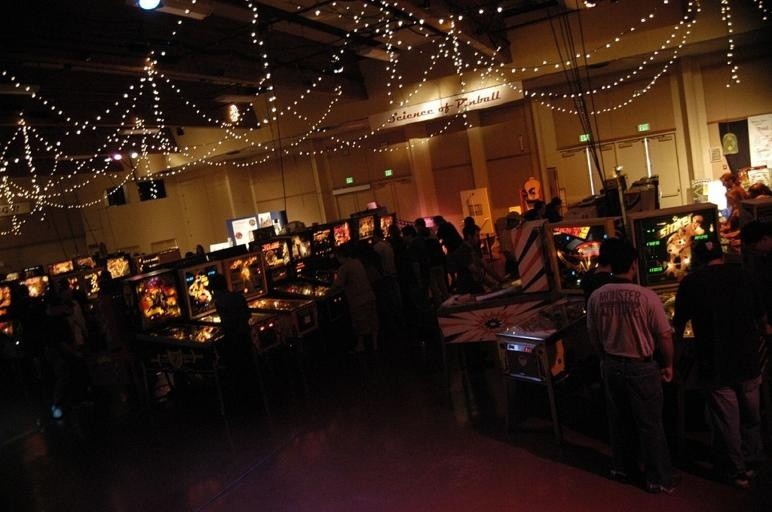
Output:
[673,239,770,487]
[743,220,770,341]
[325,216,482,352]
[498,197,564,284]
[585,238,673,490]
[209,273,252,339]
[719,173,772,237]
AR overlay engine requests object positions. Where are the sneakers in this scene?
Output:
[734,468,759,490]
[646,472,681,494]
